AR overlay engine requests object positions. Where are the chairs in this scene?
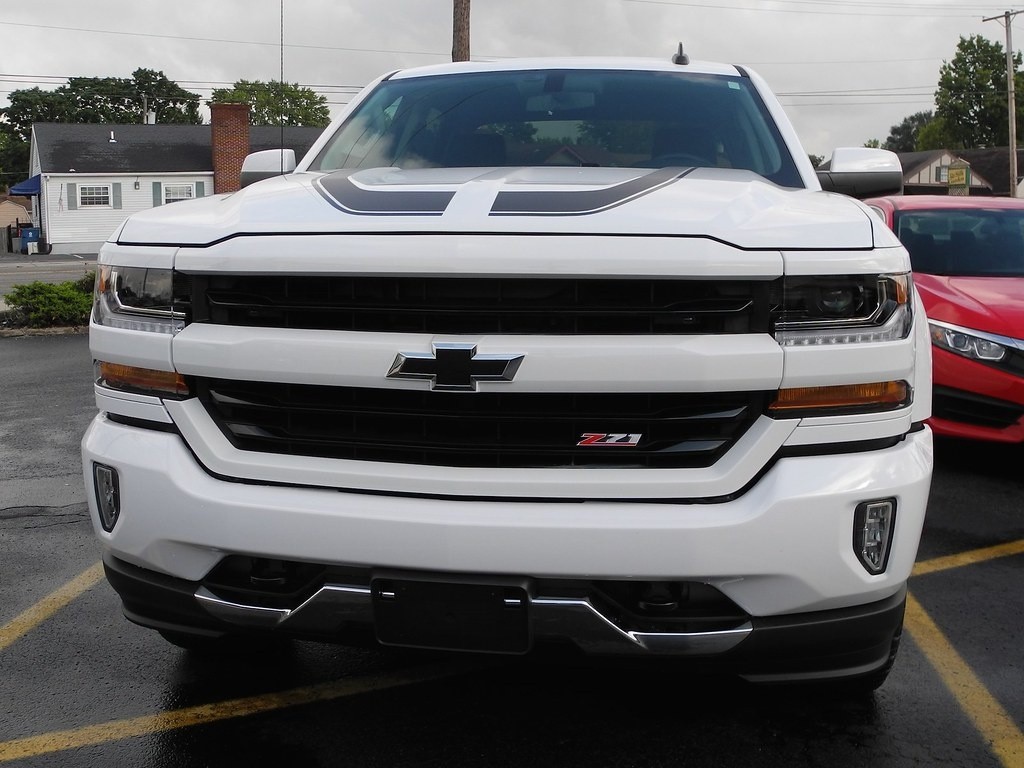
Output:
[653,127,718,166]
[444,133,506,167]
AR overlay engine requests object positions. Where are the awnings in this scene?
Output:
[9,174,41,196]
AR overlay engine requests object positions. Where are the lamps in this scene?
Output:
[135,182,140,190]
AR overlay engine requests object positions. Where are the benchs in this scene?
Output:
[903,230,1024,276]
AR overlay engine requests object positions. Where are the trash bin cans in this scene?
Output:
[21,227,39,254]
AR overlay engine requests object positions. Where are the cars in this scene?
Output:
[862,195,1024,464]
[81,56,933,691]
